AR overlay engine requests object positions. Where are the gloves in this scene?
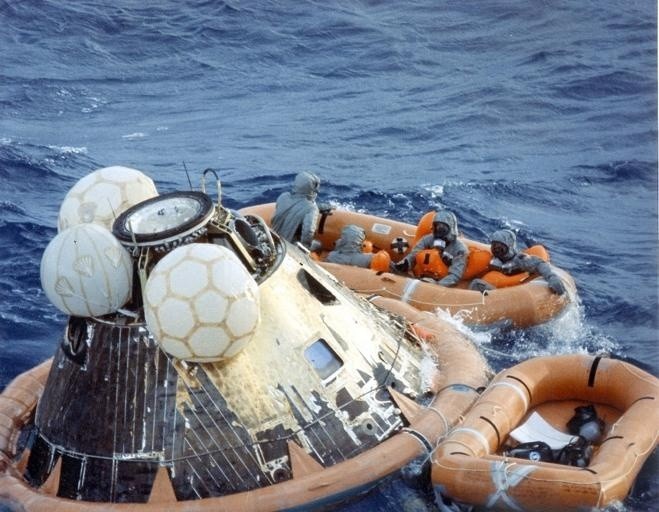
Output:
[547,276,565,295]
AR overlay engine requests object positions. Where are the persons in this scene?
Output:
[470,228,566,295]
[323,224,375,270]
[390,209,468,287]
[270,171,320,251]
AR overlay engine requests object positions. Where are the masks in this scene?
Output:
[491,247,504,257]
[434,227,446,238]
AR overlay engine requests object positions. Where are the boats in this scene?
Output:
[235,195,578,331]
[426,347,659,512]
